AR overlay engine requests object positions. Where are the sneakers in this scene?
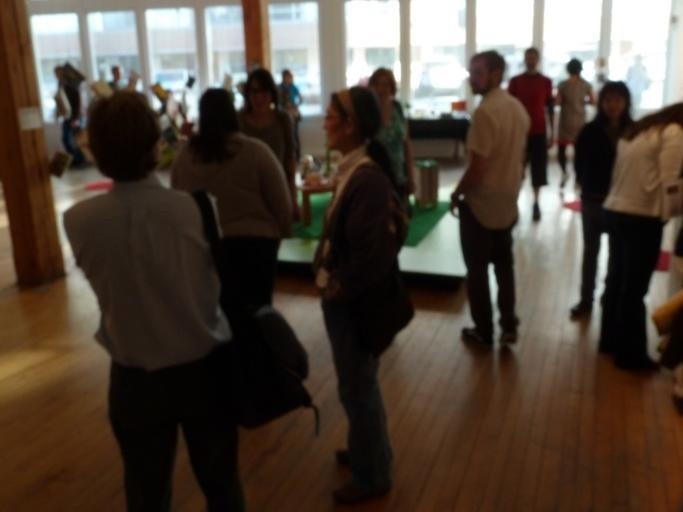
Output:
[533,205,542,219]
[336,445,356,462]
[463,326,495,350]
[559,173,570,189]
[572,300,594,318]
[502,328,519,351]
[333,484,375,509]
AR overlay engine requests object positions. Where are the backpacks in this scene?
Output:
[229,307,322,437]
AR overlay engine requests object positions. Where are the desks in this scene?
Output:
[295,172,334,226]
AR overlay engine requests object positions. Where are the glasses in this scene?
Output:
[251,86,271,95]
[324,113,348,122]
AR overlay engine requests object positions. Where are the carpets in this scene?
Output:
[284,190,449,247]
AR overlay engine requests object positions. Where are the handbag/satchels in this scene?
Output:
[393,99,416,197]
[335,182,413,352]
[198,192,304,432]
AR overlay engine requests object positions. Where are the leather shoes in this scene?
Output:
[617,356,656,372]
[599,339,612,352]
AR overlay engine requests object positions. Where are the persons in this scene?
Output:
[506,47,555,222]
[64,95,245,512]
[556,57,597,188]
[449,51,531,347]
[276,70,303,108]
[107,64,120,93]
[54,66,90,170]
[369,68,415,198]
[569,83,636,319]
[172,89,292,325]
[307,87,415,505]
[597,102,683,372]
[238,68,299,179]
